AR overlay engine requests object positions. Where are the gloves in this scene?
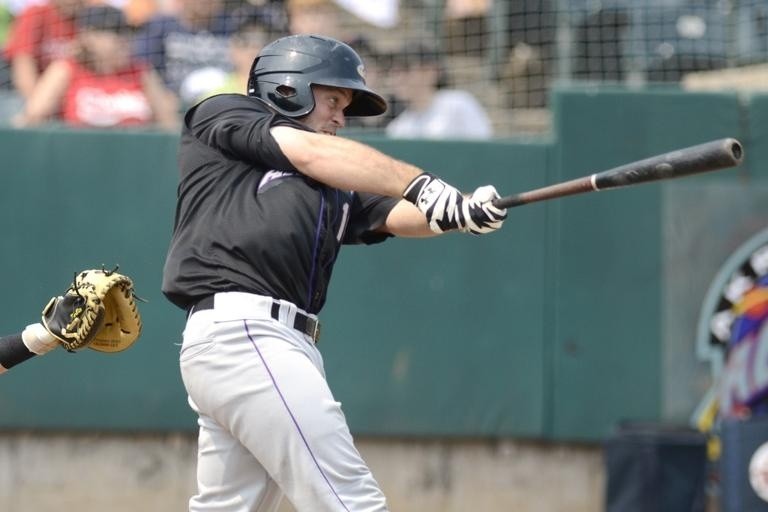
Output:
[400,171,466,235]
[464,184,508,237]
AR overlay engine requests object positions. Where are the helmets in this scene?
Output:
[247,34,388,120]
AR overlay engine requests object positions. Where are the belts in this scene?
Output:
[186,291,321,343]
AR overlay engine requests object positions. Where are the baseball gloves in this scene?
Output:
[40,263,149,353]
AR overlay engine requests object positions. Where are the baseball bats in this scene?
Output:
[491,137,744,210]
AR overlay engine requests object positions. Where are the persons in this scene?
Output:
[2,1,645,141]
[159,33,506,512]
[1,264,148,374]
